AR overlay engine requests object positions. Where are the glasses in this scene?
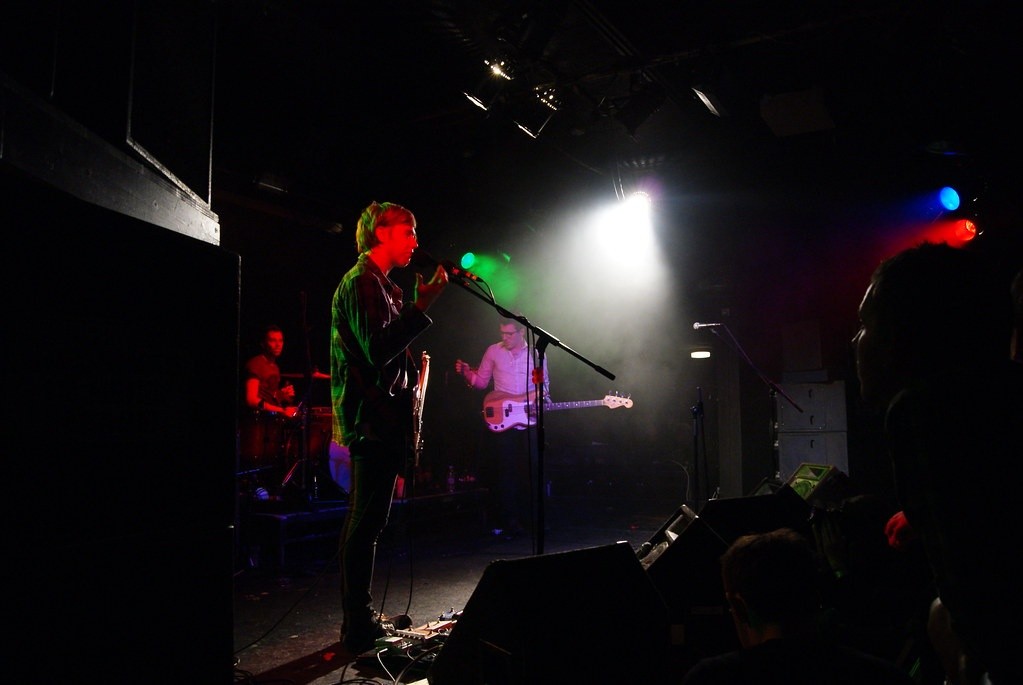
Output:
[498,330,520,338]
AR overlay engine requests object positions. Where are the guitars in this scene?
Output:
[400,350,431,474]
[482,388,634,433]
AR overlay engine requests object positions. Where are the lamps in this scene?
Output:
[460,52,518,112]
[939,172,985,213]
[511,80,566,141]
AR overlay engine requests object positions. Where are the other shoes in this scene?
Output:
[340,609,412,651]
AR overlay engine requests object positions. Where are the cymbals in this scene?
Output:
[279,371,331,381]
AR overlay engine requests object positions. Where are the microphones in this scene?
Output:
[439,259,484,283]
[693,322,725,330]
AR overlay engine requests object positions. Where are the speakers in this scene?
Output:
[425,462,858,685]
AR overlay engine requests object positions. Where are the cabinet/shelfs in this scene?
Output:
[777,383,855,484]
[770,317,829,372]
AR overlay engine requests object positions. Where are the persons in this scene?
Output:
[243,323,321,515]
[682,244,1023,685]
[330,199,450,655]
[456,318,551,540]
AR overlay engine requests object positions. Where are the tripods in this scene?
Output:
[279,381,348,502]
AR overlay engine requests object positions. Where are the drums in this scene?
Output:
[279,412,351,501]
